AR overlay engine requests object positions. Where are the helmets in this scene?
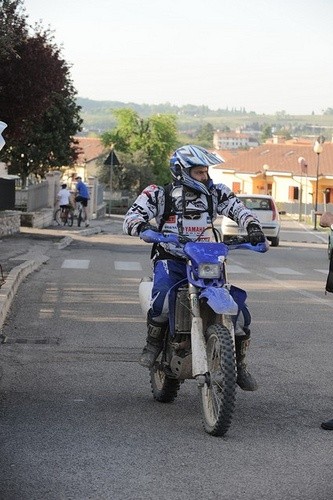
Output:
[170,143,225,196]
[75,176,82,179]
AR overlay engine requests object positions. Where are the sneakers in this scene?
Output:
[85,222,89,227]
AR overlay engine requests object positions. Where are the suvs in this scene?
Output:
[220,195,286,246]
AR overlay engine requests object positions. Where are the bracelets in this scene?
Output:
[331,224,333,231]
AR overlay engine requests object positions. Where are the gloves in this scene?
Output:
[136,222,158,235]
[245,223,265,246]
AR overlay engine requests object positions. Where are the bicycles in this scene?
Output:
[54,205,73,227]
[77,202,85,227]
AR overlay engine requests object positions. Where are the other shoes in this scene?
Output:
[321,419,333,430]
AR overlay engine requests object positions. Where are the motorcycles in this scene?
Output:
[137,227,270,437]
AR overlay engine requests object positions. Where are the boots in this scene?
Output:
[235,327,258,391]
[139,308,169,368]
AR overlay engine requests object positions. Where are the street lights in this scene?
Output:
[297,156,306,222]
[313,139,324,231]
[263,164,269,195]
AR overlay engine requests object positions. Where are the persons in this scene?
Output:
[57,183,72,221]
[320,211,333,430]
[72,177,89,228]
[123,146,266,391]
